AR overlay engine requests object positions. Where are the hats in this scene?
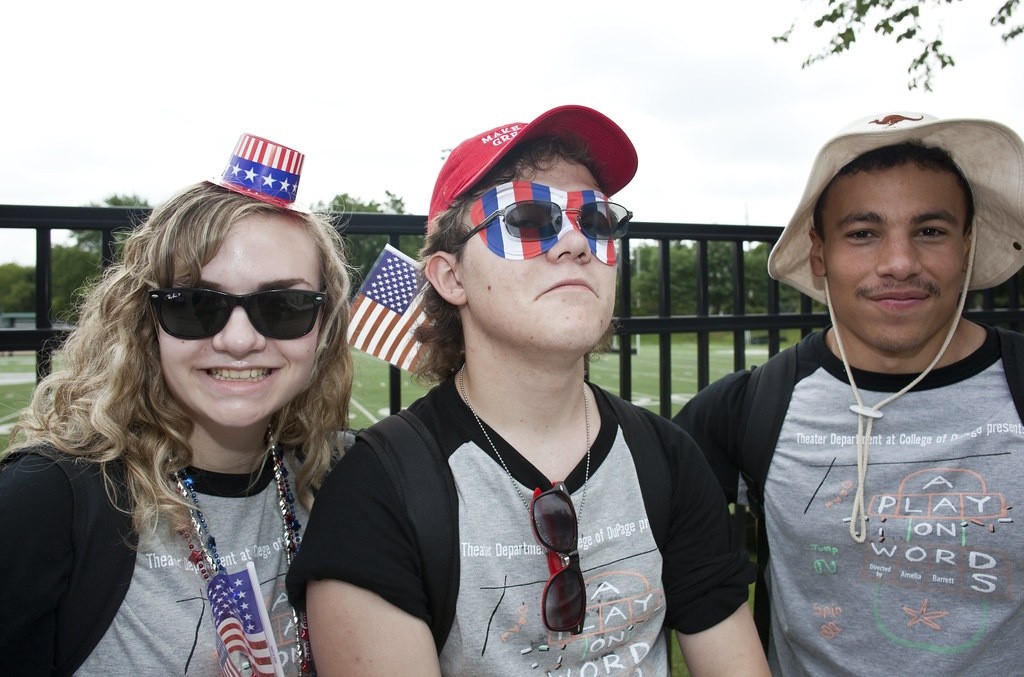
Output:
[767,111,1024,304]
[427,104,638,235]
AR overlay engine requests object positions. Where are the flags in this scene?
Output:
[346,251,437,371]
[207,562,275,677]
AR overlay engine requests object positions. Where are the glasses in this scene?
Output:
[146,288,326,341]
[459,199,633,242]
[530,481,586,635]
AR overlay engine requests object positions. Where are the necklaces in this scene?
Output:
[168,426,315,677]
[460,363,590,567]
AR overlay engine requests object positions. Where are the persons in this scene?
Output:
[669,116,1024,677]
[1,134,365,677]
[284,103,772,677]
